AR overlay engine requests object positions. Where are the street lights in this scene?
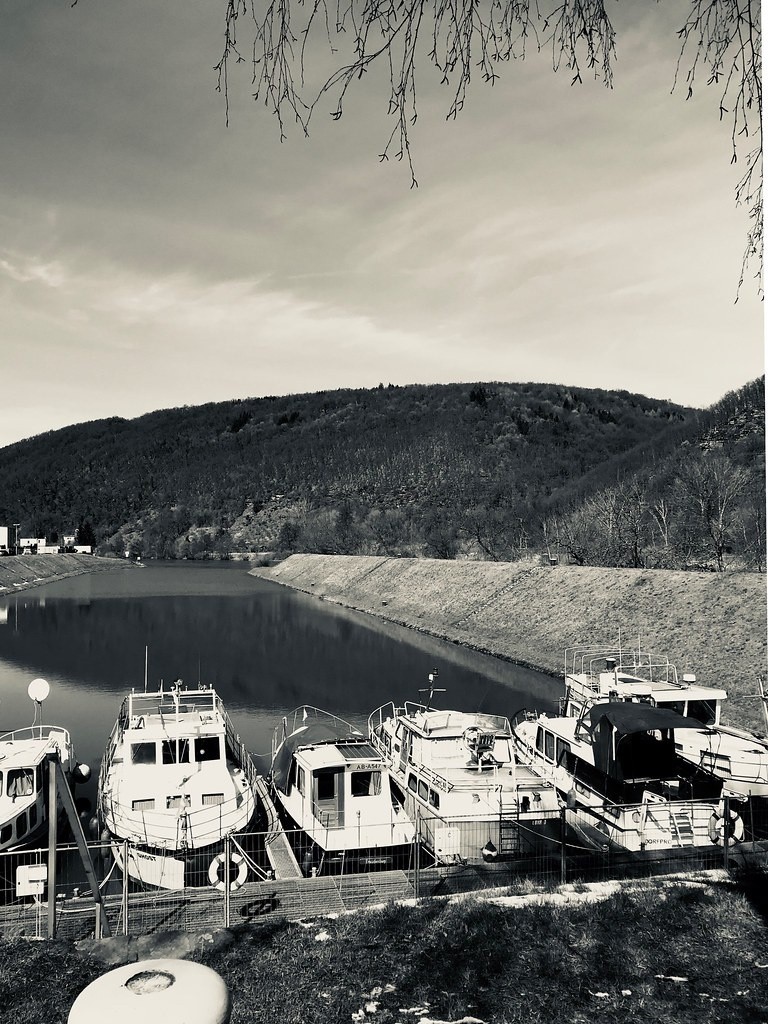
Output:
[14,523,20,555]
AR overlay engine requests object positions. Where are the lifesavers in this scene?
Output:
[207,852,249,893]
[707,810,745,845]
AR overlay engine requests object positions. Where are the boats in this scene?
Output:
[271,706,421,874]
[0,678,77,850]
[98,646,260,892]
[558,624,768,804]
[367,702,562,865]
[515,690,746,852]
